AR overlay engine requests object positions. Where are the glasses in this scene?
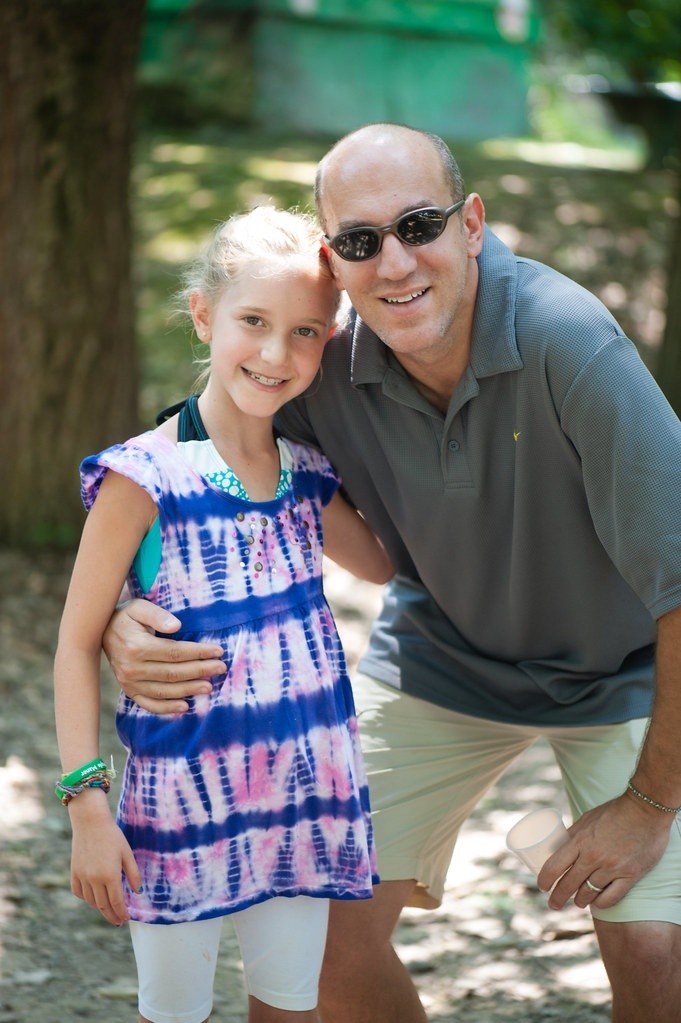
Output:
[322,200,466,262]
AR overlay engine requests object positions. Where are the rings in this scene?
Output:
[585,878,604,894]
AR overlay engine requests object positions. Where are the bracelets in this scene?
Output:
[54,758,112,807]
[628,782,681,812]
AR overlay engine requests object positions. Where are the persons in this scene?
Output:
[101,125,681,1023]
[54,205,401,1022]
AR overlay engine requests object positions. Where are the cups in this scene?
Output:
[506,808,579,898]
